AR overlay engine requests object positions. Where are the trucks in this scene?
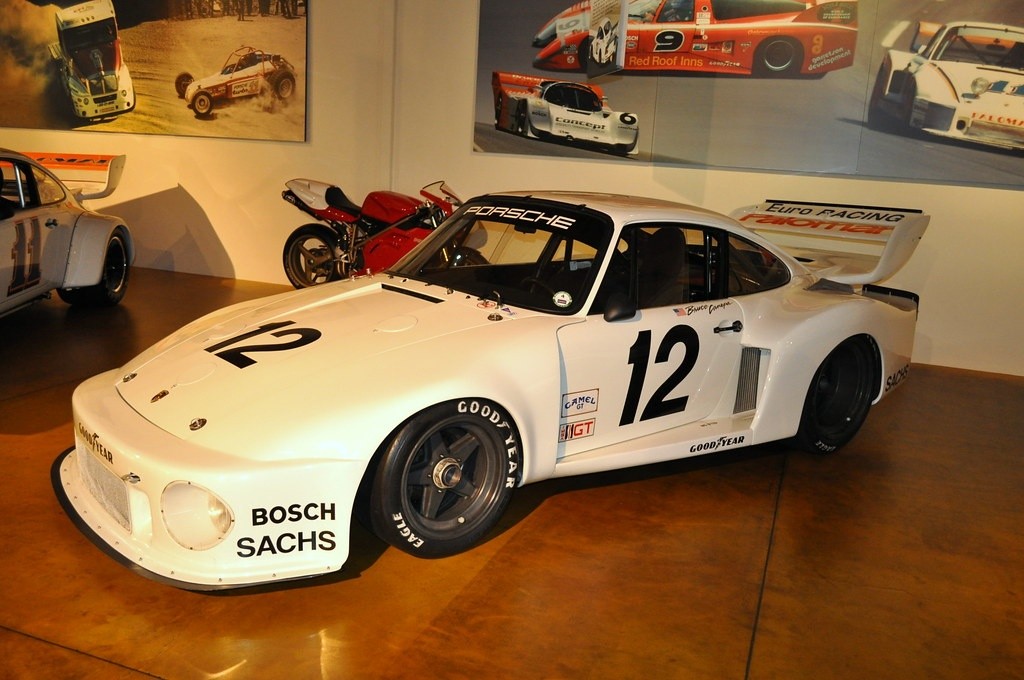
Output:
[48,0,136,120]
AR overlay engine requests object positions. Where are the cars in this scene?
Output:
[491,71,639,156]
[0,147,135,317]
[531,0,858,80]
[868,20,1024,150]
[175,46,295,117]
[51,189,931,590]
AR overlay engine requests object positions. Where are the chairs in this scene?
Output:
[624,227,686,309]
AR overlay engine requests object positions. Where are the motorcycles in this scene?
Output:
[282,179,491,290]
